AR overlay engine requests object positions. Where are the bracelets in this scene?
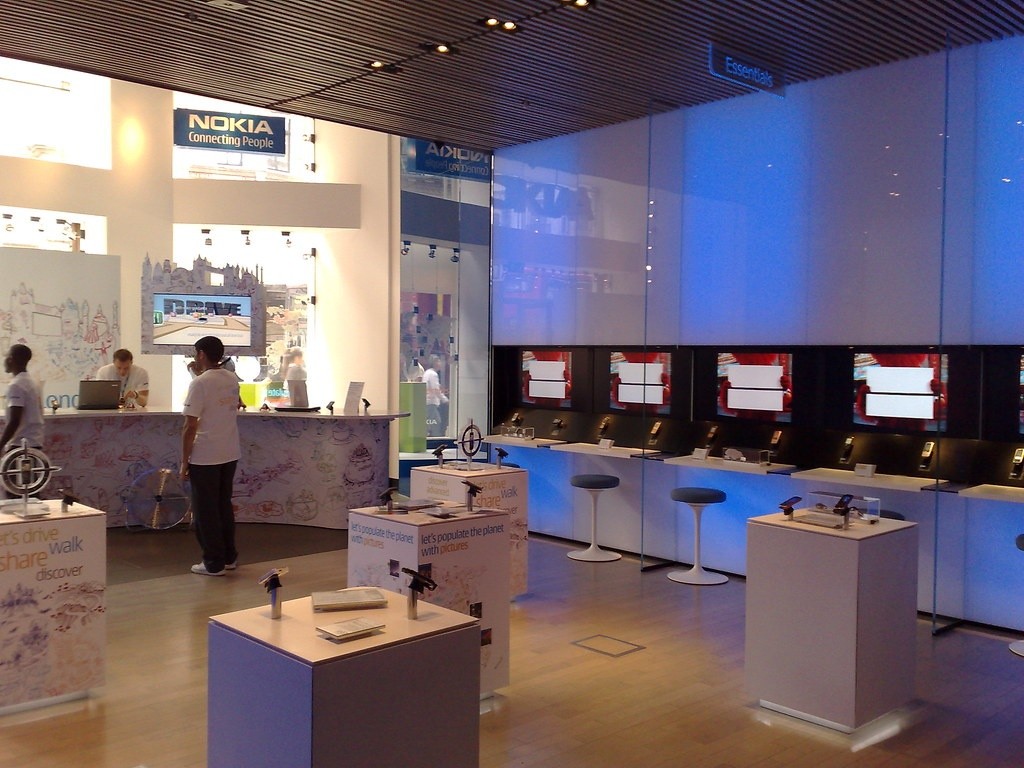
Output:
[134,391,138,399]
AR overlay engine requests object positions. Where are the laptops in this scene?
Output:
[76,380,121,410]
[276,407,321,411]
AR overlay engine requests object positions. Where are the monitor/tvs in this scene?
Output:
[153,293,251,348]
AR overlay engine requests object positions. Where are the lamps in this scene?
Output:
[401,247,410,256]
[428,250,436,258]
[204,236,292,249]
[450,255,459,262]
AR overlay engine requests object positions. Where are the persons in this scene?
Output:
[284,346,307,382]
[96,348,150,407]
[421,354,448,437]
[178,335,246,576]
[0,343,46,502]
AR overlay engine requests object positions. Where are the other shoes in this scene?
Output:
[191,560,236,575]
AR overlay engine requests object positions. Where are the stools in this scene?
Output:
[566,473,622,562]
[665,486,730,586]
[1008,534,1024,658]
[859,508,905,521]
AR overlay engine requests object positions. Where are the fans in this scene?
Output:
[124,467,194,532]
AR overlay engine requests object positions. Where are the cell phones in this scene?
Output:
[771,430,782,444]
[511,413,519,421]
[432,445,448,454]
[462,479,482,491]
[834,494,853,510]
[258,569,278,583]
[553,418,559,424]
[326,401,335,409]
[601,417,609,424]
[708,426,718,437]
[362,398,370,405]
[402,568,435,587]
[779,496,802,508]
[651,422,661,435]
[921,442,935,458]
[1013,448,1024,466]
[840,436,854,448]
[379,487,398,498]
[58,489,80,503]
